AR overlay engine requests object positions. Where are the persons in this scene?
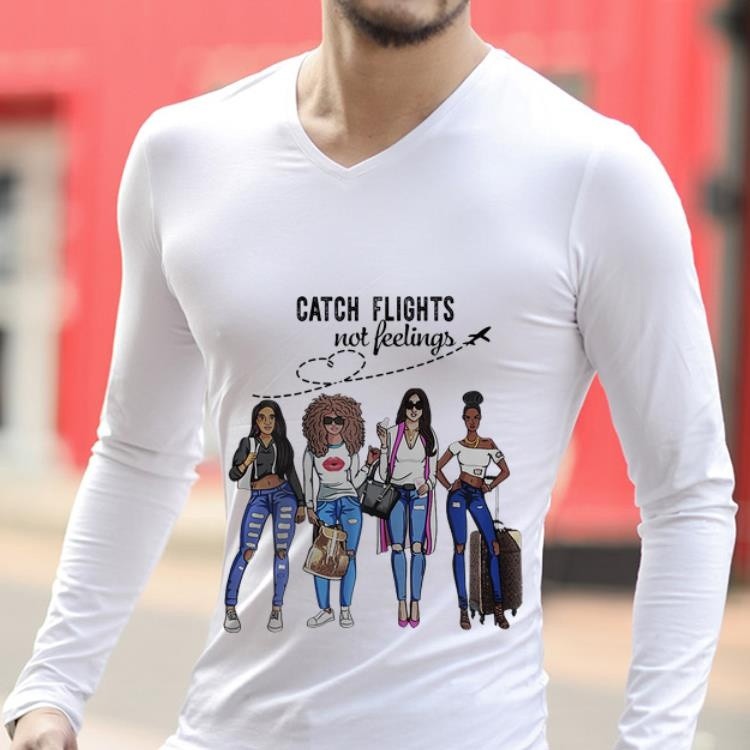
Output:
[0,0,740,750]
